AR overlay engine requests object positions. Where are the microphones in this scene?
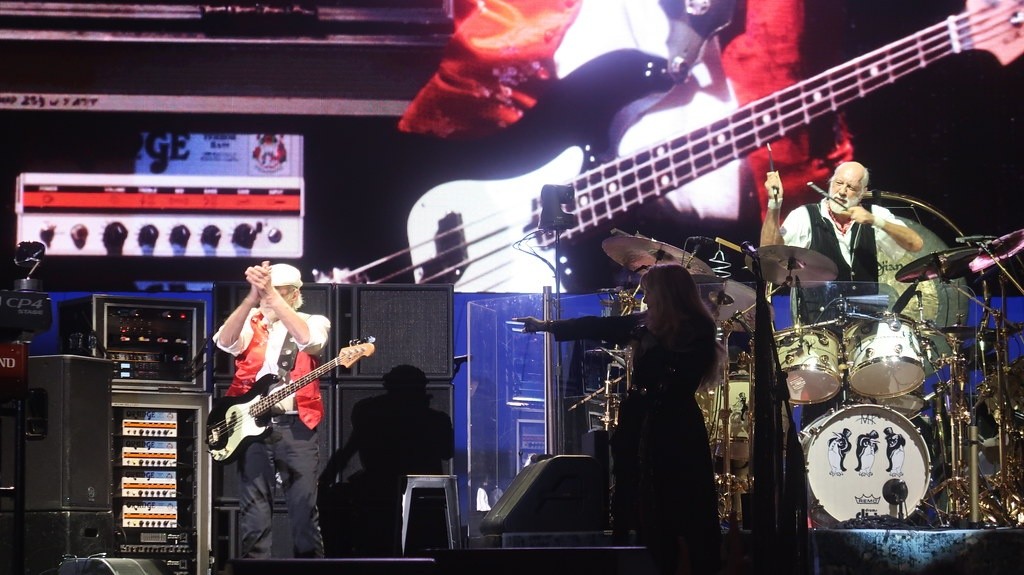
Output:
[955,235,998,242]
[889,480,906,499]
[689,236,714,245]
[454,354,473,364]
[892,282,918,315]
[741,241,760,259]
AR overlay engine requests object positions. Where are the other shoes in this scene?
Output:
[808,498,838,530]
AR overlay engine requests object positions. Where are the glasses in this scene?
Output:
[258,289,296,300]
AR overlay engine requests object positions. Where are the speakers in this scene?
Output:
[229,281,454,550]
[478,454,604,537]
[0,354,176,575]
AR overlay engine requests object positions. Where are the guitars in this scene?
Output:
[406,0,1024,293]
[206,335,377,465]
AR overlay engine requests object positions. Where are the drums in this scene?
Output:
[707,374,755,462]
[844,385,925,421]
[842,312,926,398]
[798,403,932,529]
[772,327,842,405]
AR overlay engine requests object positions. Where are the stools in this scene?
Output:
[400,475,460,557]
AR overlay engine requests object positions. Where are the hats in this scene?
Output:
[269,264,303,289]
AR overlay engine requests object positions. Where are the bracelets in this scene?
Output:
[872,216,885,229]
[768,198,783,208]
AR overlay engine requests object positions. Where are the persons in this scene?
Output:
[213,260,331,560]
[516,264,729,575]
[397,1,853,292]
[760,162,923,429]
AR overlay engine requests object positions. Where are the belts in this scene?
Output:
[259,414,300,424]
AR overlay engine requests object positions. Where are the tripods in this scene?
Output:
[915,270,1024,527]
[712,279,792,523]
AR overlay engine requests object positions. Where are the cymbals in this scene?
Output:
[895,246,981,284]
[921,323,997,339]
[942,228,1024,279]
[602,236,714,285]
[690,273,775,332]
[745,245,838,288]
